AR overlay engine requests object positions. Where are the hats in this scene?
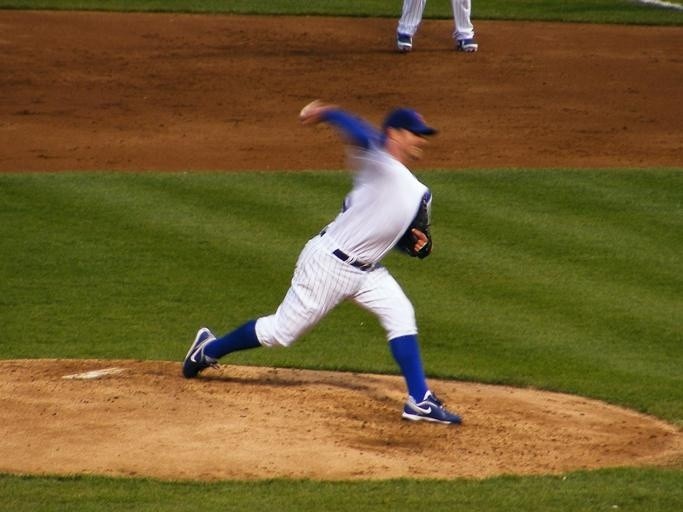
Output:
[384,107,438,135]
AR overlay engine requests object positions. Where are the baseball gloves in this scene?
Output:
[397,196,431,258]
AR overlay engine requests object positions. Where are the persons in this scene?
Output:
[396,0,478,54]
[180,98,464,429]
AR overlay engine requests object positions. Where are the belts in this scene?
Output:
[320,230,371,270]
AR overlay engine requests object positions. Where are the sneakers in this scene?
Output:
[395,30,413,51]
[182,327,220,379]
[401,389,462,425]
[457,36,479,53]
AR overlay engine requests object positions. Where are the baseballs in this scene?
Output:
[301,99,321,115]
